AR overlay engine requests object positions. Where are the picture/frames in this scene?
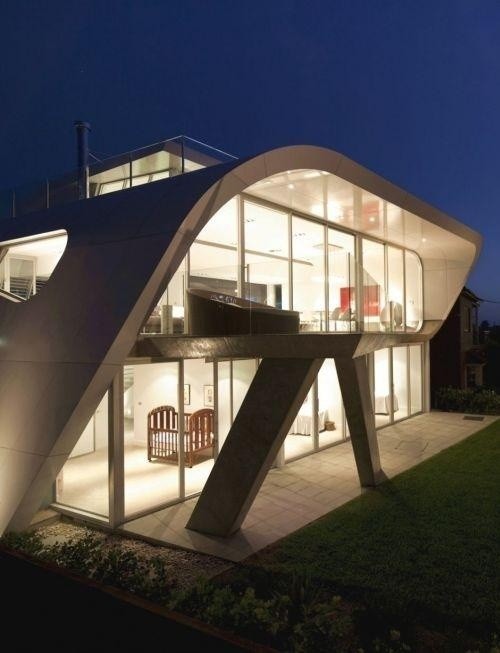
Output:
[176,382,191,405]
[202,383,216,407]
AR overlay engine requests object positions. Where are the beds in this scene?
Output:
[146,401,219,468]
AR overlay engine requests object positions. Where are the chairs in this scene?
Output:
[185,286,302,336]
[315,303,357,331]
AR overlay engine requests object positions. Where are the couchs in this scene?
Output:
[144,312,184,333]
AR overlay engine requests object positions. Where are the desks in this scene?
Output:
[375,389,400,415]
[286,409,329,436]
